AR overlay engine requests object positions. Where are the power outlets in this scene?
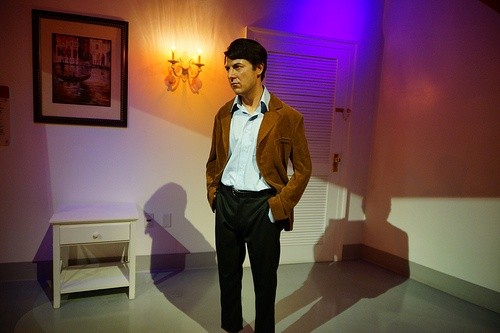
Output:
[144,212,154,227]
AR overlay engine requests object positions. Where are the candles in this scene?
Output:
[171,45,175,60]
[198,50,201,63]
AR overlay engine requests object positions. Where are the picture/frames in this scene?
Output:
[31,9,130,128]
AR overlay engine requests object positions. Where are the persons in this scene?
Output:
[206,39,312,332]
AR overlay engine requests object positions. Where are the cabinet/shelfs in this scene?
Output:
[50,202,139,308]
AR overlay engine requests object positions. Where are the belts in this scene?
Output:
[232,189,273,197]
